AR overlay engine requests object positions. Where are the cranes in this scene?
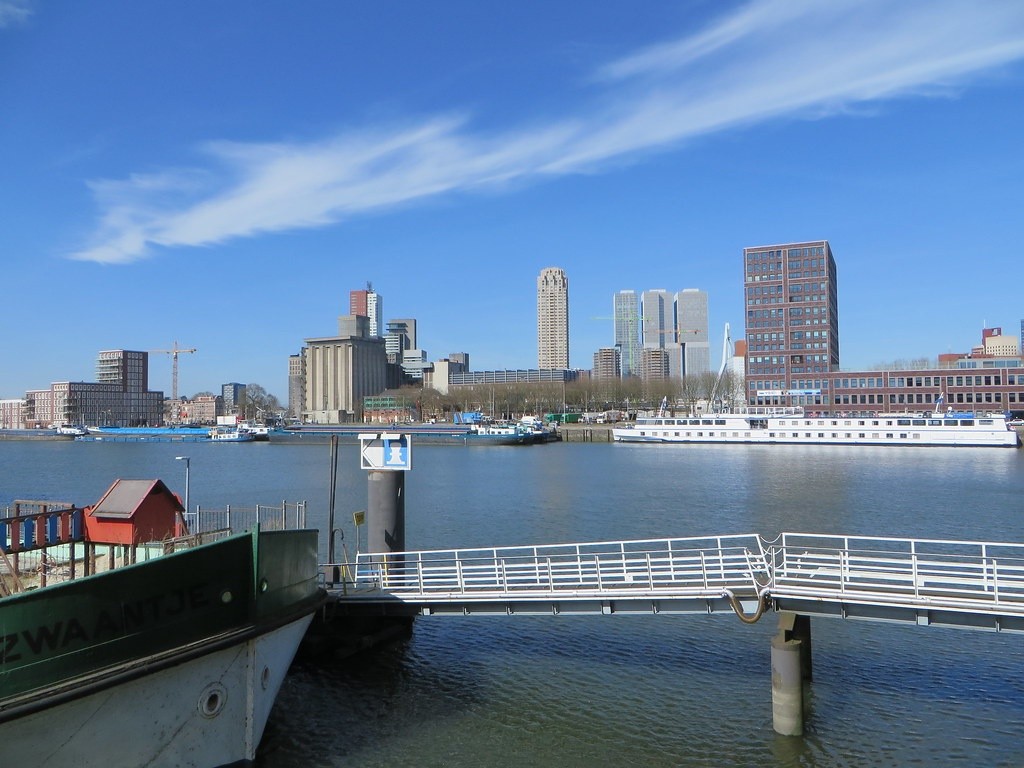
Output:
[145,339,197,400]
[643,319,703,377]
[587,302,654,379]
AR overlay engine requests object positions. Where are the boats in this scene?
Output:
[0,472,338,768]
[611,392,1019,447]
[56,408,561,445]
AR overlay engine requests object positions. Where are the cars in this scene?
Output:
[1006,419,1024,426]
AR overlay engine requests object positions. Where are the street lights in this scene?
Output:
[174,456,191,521]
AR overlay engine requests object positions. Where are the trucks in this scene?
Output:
[596,410,622,424]
[559,412,582,423]
[577,412,601,424]
[544,413,562,422]
